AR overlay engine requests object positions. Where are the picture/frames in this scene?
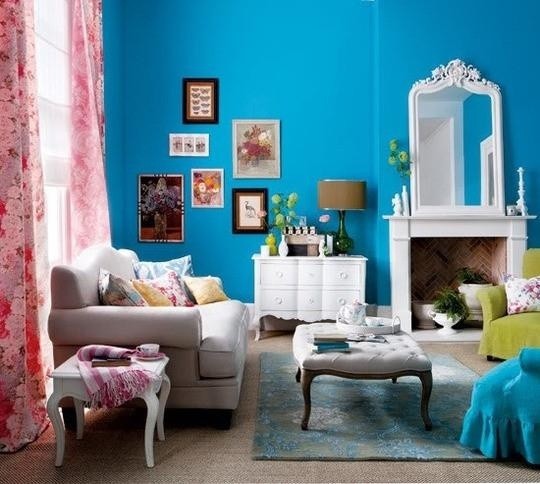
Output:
[232,119,279,178]
[169,133,210,156]
[191,168,225,208]
[183,77,218,124]
[138,174,185,243]
[232,188,268,234]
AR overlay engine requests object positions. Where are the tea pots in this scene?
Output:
[340,297,367,326]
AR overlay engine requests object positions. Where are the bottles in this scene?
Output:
[278,234,289,256]
[288,226,316,235]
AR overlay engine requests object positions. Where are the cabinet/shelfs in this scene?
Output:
[251,253,368,342]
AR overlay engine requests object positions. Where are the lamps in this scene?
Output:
[318,179,366,256]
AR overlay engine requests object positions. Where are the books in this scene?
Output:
[91,356,131,368]
[312,333,352,353]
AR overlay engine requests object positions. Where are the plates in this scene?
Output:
[134,353,165,361]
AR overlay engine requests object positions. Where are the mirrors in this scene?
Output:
[407,58,506,216]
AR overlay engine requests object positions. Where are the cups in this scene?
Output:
[507,205,518,215]
[261,245,270,257]
[135,343,160,356]
[364,316,382,326]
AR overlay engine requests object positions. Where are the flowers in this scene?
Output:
[388,139,412,185]
[318,214,330,234]
[256,192,298,235]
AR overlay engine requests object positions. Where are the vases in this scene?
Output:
[402,185,410,217]
[265,238,289,257]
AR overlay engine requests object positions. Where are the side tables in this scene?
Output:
[47,351,171,470]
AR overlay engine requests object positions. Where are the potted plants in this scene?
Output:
[430,262,494,336]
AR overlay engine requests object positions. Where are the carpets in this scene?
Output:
[251,352,506,462]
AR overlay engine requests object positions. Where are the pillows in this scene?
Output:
[181,276,230,305]
[131,280,171,307]
[131,254,196,304]
[149,270,195,307]
[98,267,149,306]
[504,276,539,315]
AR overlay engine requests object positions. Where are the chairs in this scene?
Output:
[475,248,540,361]
[48,245,251,430]
[460,347,540,465]
